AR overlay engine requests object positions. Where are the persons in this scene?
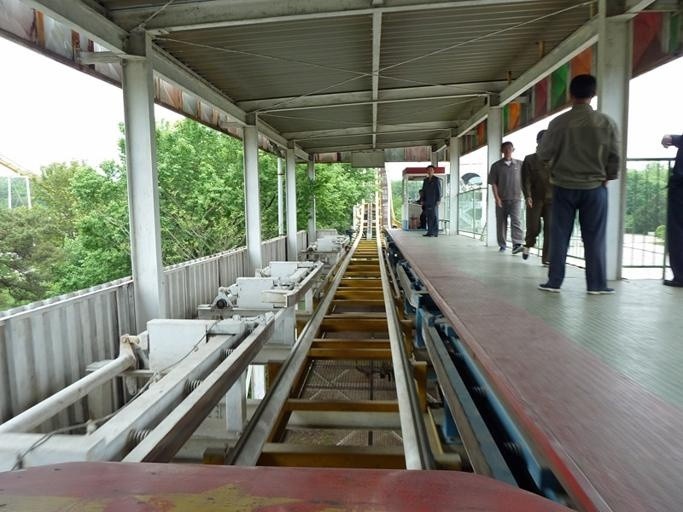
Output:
[521,129,550,267]
[413,189,426,229]
[536,74,622,295]
[422,165,443,237]
[487,141,524,254]
[660,132,683,287]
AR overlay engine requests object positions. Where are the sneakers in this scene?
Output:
[664,279,682,287]
[499,244,529,260]
[538,284,561,292]
[423,233,438,237]
[587,287,615,294]
[542,261,550,266]
